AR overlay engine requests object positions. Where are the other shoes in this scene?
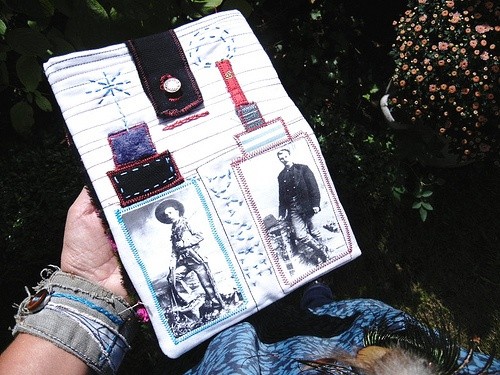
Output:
[297,267,337,318]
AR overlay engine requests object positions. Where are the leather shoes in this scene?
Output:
[318,245,329,262]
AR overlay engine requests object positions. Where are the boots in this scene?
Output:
[212,297,223,310]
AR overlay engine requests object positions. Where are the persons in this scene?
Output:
[272,148,332,263]
[18,178,499,375]
[140,197,226,319]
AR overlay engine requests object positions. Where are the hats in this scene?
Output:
[155,198,185,223]
